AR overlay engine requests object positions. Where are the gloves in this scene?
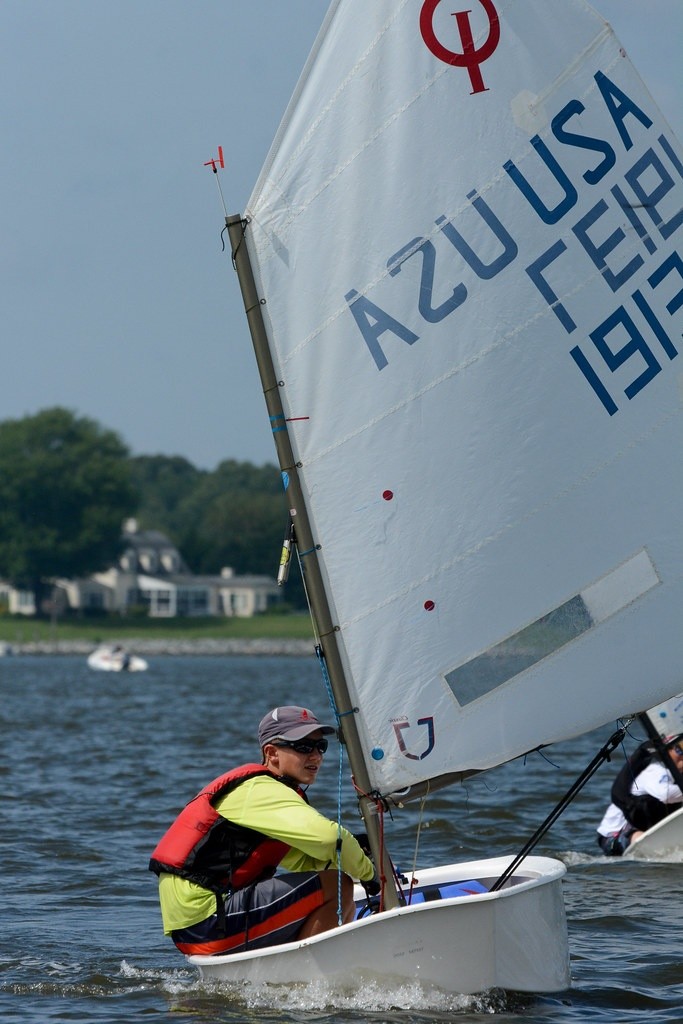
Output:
[360,869,381,896]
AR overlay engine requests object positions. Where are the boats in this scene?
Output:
[83,640,150,675]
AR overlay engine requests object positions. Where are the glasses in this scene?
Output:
[674,742,683,756]
[268,737,328,753]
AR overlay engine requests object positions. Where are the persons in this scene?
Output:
[596,733,683,857]
[148,705,382,957]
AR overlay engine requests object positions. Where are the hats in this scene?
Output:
[258,705,335,747]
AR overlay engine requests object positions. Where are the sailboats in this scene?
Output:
[163,3,682,1007]
[607,696,682,864]
